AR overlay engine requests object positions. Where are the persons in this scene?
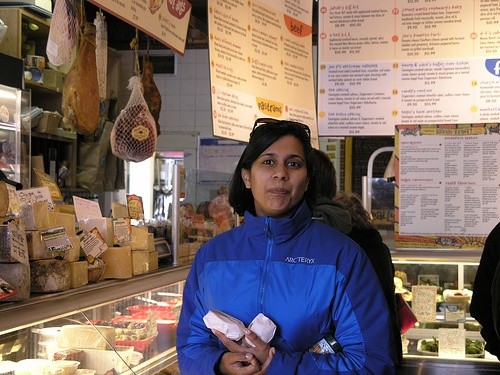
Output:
[303,148,405,375]
[469,222,500,362]
[174,121,394,375]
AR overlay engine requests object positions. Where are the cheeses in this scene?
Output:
[0,181,158,302]
[0,325,136,375]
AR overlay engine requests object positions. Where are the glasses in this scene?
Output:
[249,118,311,146]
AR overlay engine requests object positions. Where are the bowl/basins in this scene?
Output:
[405,295,443,310]
[16,304,179,375]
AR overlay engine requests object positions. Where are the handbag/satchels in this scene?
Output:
[395,293,417,335]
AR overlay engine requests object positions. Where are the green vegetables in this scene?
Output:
[421,336,484,355]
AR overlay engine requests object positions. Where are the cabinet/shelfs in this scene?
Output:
[0,0,105,217]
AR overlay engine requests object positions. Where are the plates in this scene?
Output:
[396,289,410,294]
[417,337,486,357]
[443,288,473,303]
